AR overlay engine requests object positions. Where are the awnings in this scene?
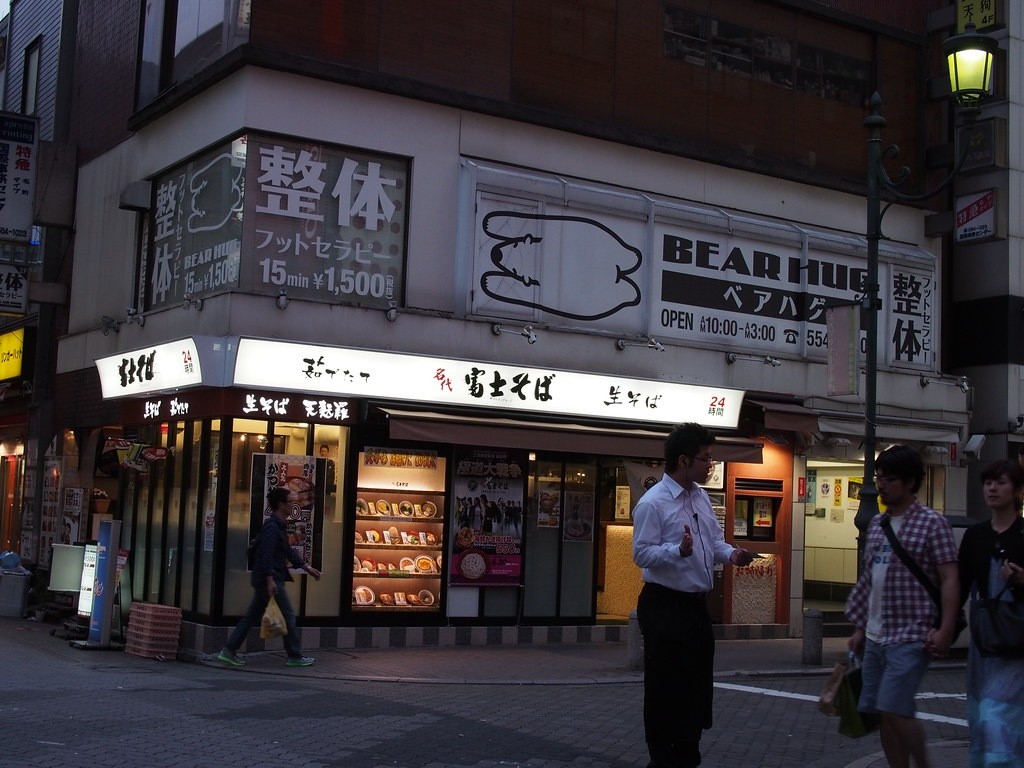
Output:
[378,406,763,463]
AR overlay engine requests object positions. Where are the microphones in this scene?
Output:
[693,513,698,520]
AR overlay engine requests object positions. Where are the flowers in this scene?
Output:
[93,487,110,500]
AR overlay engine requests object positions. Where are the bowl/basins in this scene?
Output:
[391,536,400,544]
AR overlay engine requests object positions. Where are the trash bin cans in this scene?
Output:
[0,567,32,619]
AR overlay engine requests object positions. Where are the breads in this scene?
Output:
[263,615,288,636]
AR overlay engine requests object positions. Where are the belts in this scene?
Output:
[646,582,706,600]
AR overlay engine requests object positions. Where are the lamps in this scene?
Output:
[919,375,969,392]
[100,314,119,336]
[492,320,537,345]
[387,301,398,323]
[182,292,204,312]
[616,337,666,353]
[275,286,290,310]
[125,308,145,328]
[726,353,782,367]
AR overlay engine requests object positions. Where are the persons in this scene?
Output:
[319,445,335,496]
[631,424,753,768]
[218,488,321,666]
[41,476,59,562]
[455,494,522,538]
[844,446,959,768]
[926,461,1024,768]
[61,517,71,544]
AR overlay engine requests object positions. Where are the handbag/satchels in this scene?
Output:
[259,596,288,639]
[932,608,969,645]
[969,571,1024,660]
[815,648,880,739]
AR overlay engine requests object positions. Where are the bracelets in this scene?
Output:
[305,566,312,571]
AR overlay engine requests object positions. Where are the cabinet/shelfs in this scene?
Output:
[353,487,444,611]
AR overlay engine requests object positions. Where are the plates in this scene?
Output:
[355,527,438,544]
[354,585,434,606]
[456,548,491,580]
[563,518,592,540]
[353,555,436,572]
[356,497,437,517]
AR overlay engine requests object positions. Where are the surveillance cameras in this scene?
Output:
[962,435,986,457]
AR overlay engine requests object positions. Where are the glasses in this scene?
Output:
[287,500,294,505]
[684,453,712,462]
[875,476,903,484]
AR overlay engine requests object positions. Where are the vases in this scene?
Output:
[92,499,113,514]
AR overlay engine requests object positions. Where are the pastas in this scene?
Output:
[461,552,486,579]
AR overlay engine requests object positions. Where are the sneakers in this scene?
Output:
[287,655,316,666]
[218,647,247,666]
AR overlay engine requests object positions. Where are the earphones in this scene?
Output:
[683,459,686,465]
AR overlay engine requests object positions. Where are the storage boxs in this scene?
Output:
[127,603,182,658]
